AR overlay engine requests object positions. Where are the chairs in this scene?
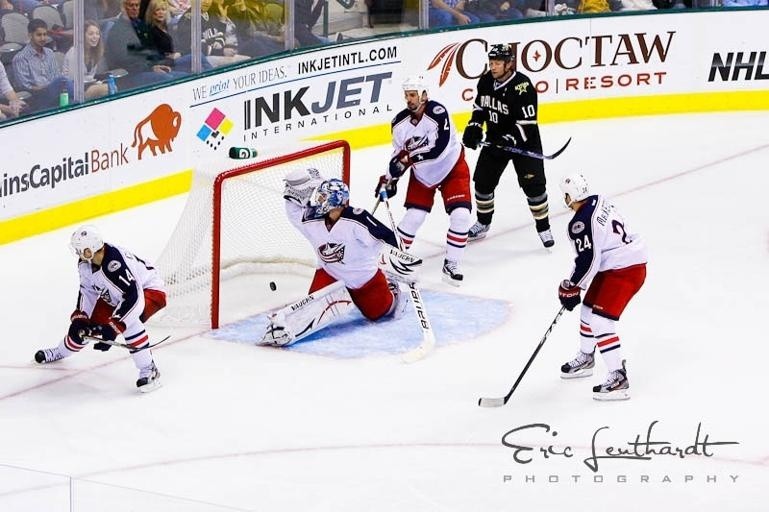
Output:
[0,0,190,86]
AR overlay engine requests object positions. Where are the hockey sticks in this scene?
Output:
[380,188,436,365]
[478,139,570,160]
[83,335,172,351]
[479,305,565,407]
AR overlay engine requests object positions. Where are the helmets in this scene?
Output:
[313,178,349,219]
[559,174,591,207]
[403,72,432,102]
[488,43,512,61]
[69,224,104,261]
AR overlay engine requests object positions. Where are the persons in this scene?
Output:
[374,72,473,285]
[34,223,173,394]
[0,1,344,125]
[460,43,554,248]
[558,172,646,399]
[255,165,426,348]
[362,0,769,33]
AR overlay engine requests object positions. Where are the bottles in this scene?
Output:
[105,73,119,98]
[59,78,69,107]
[229,147,258,162]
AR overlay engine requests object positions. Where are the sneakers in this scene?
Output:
[34,346,67,364]
[441,258,463,281]
[467,220,489,238]
[137,359,160,388]
[538,227,554,248]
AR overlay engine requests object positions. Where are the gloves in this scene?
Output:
[374,175,397,202]
[389,151,413,178]
[497,134,516,151]
[92,317,126,352]
[558,280,580,312]
[69,310,94,345]
[462,122,482,150]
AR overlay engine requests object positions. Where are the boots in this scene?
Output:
[592,359,629,393]
[561,347,597,373]
